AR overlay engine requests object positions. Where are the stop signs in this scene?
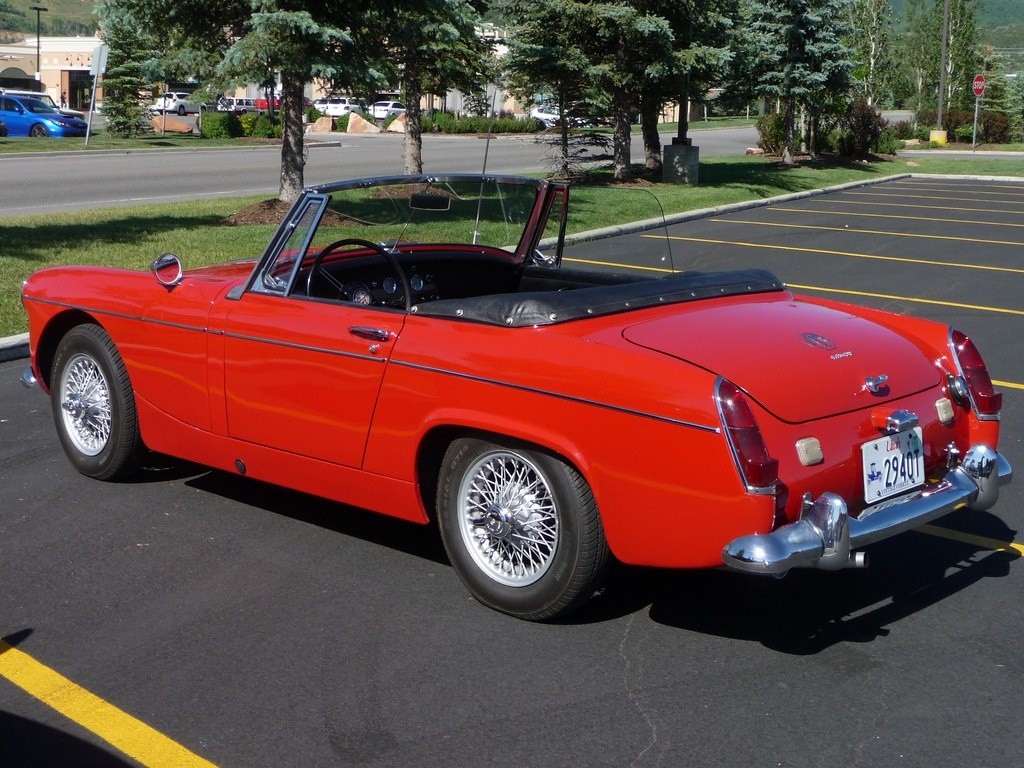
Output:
[972,74,987,97]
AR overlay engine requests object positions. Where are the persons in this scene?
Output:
[85,95,89,109]
[60,92,67,109]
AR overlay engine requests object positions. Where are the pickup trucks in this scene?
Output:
[254,94,315,117]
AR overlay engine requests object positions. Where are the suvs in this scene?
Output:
[216,97,258,116]
[156,92,207,117]
[0,87,85,121]
[529,104,575,129]
[313,96,369,118]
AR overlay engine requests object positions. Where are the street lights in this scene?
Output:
[29,6,48,92]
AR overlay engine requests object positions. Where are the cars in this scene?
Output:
[0,94,92,139]
[369,101,406,120]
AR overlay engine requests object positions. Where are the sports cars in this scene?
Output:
[17,173,1015,624]
